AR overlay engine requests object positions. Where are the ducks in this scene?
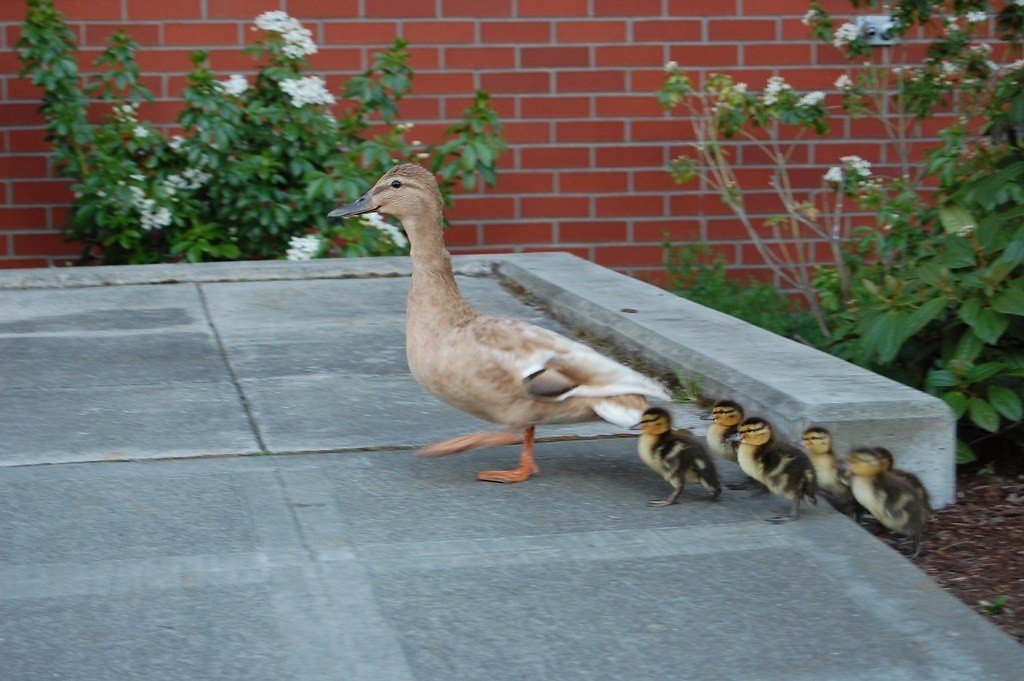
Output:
[326,162,944,556]
